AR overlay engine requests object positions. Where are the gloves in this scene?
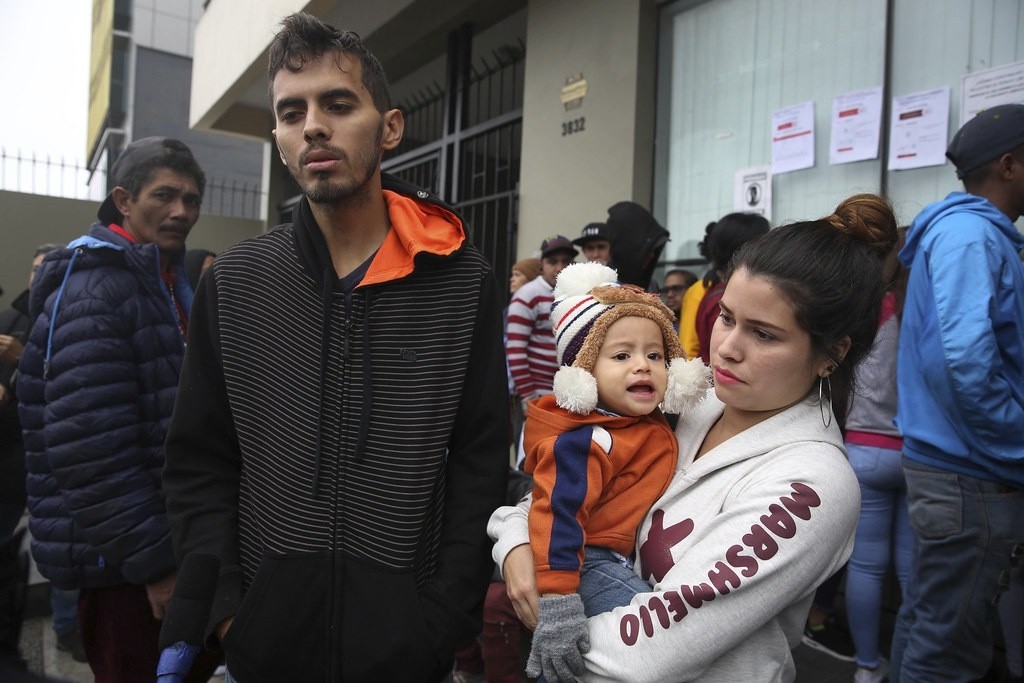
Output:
[525,592,592,683]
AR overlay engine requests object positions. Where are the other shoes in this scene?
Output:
[54,634,72,653]
[72,636,88,663]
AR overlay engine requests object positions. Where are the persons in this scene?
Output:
[845,225,917,682]
[799,563,858,663]
[524,283,682,683]
[184,247,216,293]
[502,255,544,459]
[664,269,698,333]
[1,245,67,682]
[898,103,1024,683]
[696,212,769,368]
[484,218,861,682]
[18,137,224,682]
[571,223,608,268]
[680,221,716,360]
[507,235,575,473]
[166,12,511,682]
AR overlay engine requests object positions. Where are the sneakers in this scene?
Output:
[854,658,890,683]
[801,615,858,662]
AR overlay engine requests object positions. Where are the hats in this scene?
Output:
[548,259,714,418]
[944,103,1024,180]
[513,258,544,282]
[541,234,579,257]
[97,137,198,227]
[570,220,610,247]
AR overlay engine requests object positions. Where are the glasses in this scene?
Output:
[658,285,687,296]
